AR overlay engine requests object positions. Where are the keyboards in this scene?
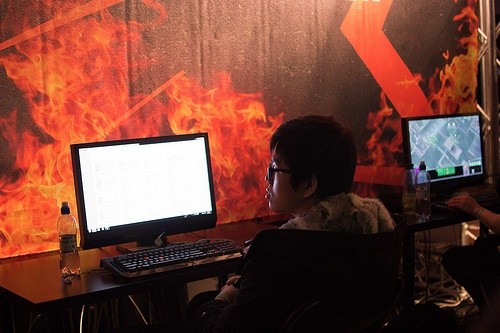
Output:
[99,239,246,280]
[430,191,500,216]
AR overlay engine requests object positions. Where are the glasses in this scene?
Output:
[266,161,297,182]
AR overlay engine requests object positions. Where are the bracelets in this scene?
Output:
[216,295,231,303]
[475,207,487,218]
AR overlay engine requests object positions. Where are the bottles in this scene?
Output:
[57,202,80,284]
[402,163,417,216]
[415,161,431,219]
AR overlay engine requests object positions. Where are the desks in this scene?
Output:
[0,180,500,333]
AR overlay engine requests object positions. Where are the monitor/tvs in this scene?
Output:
[401,112,488,200]
[69,131,217,253]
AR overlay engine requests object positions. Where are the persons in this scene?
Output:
[442,194,500,316]
[193,115,399,333]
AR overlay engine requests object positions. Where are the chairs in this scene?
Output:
[142,225,409,333]
